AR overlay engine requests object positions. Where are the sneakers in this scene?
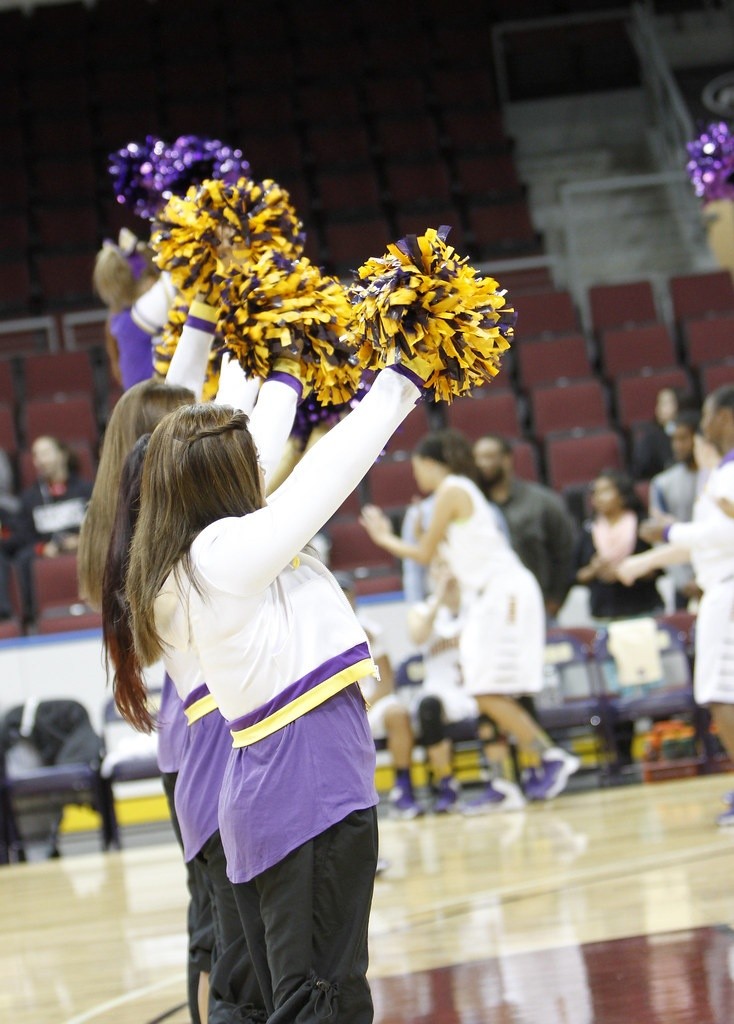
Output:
[383,749,581,814]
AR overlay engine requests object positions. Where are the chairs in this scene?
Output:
[0,0,734,864]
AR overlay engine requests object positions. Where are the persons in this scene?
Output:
[0,433,96,638]
[94,218,178,392]
[76,227,236,1024]
[101,301,305,1024]
[337,382,734,828]
[126,359,429,1024]
